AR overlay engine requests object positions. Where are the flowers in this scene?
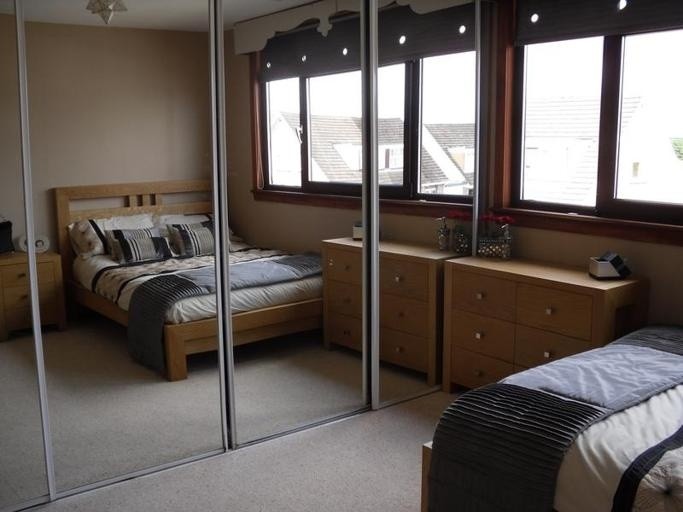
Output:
[444,208,471,228]
[480,210,513,237]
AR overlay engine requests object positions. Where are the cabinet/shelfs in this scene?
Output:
[440,259,652,399]
[319,229,467,386]
[1,241,66,342]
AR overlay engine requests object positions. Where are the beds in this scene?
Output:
[423,326,683,512]
[51,179,326,382]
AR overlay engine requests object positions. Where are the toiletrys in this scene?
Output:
[434,215,450,251]
[498,222,514,259]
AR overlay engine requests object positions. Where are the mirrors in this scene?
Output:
[216,1,377,448]
[0,1,51,510]
[370,1,480,404]
[18,0,227,499]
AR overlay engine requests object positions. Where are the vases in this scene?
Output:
[449,229,471,254]
[479,237,511,258]
